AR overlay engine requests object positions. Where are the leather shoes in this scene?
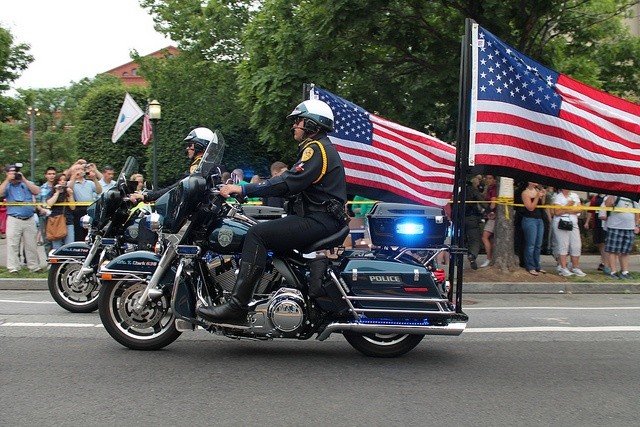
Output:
[536,268,546,273]
[468,254,477,270]
[528,270,538,275]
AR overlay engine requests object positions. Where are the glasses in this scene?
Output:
[293,117,300,124]
[187,143,192,148]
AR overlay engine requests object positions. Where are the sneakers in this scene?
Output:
[558,267,573,276]
[8,269,18,273]
[597,264,604,271]
[567,262,573,269]
[30,267,42,272]
[609,272,623,280]
[571,268,586,276]
[620,271,635,279]
[604,266,610,273]
[556,264,561,270]
[480,258,493,267]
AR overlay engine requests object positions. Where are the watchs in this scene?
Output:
[635,224,640,228]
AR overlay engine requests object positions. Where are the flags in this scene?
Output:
[465,22,640,202]
[140,100,152,145]
[111,91,144,143]
[309,82,462,207]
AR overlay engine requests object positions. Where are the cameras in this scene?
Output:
[13,162,24,180]
[83,164,90,167]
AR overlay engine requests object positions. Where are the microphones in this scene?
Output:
[184,148,194,152]
[289,126,313,134]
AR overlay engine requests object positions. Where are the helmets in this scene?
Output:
[184,127,218,152]
[286,100,334,132]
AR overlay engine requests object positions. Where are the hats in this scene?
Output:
[6,165,15,172]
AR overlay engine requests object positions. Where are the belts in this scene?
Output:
[13,215,32,220]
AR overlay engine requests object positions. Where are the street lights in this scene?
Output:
[147,98,162,193]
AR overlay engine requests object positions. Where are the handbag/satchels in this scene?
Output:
[46,215,67,241]
[558,219,572,231]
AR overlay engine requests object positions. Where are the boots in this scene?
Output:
[198,262,264,325]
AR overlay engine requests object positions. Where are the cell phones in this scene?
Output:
[80,172,90,176]
[59,186,68,193]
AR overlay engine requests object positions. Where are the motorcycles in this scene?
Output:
[97,130,469,358]
[45,156,286,312]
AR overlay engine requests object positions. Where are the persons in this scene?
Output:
[66,163,102,242]
[598,194,612,275]
[434,195,452,264]
[550,187,574,275]
[69,158,104,182]
[521,180,547,275]
[464,180,496,269]
[261,161,289,207]
[0,164,44,273]
[197,99,355,323]
[45,172,76,251]
[604,194,640,279]
[584,193,607,271]
[543,185,554,222]
[513,178,528,267]
[230,168,244,184]
[35,167,57,270]
[129,173,144,192]
[249,174,260,183]
[30,181,44,245]
[552,188,586,276]
[479,174,498,267]
[60,169,70,181]
[221,171,230,184]
[93,165,117,213]
[130,127,219,251]
